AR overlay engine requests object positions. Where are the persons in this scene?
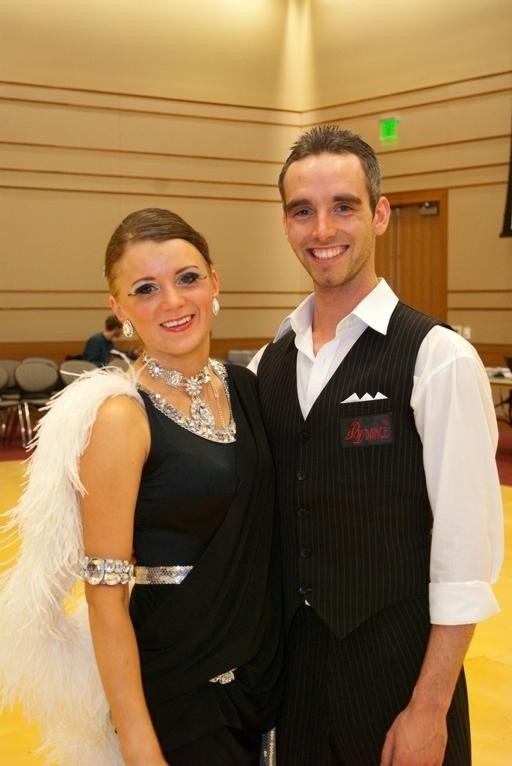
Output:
[1,207,287,766]
[243,123,506,766]
[85,314,125,367]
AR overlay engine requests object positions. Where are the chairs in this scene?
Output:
[0,358,134,450]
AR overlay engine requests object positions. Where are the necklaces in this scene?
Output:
[140,350,216,432]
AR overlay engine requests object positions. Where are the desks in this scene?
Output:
[490,377,511,423]
